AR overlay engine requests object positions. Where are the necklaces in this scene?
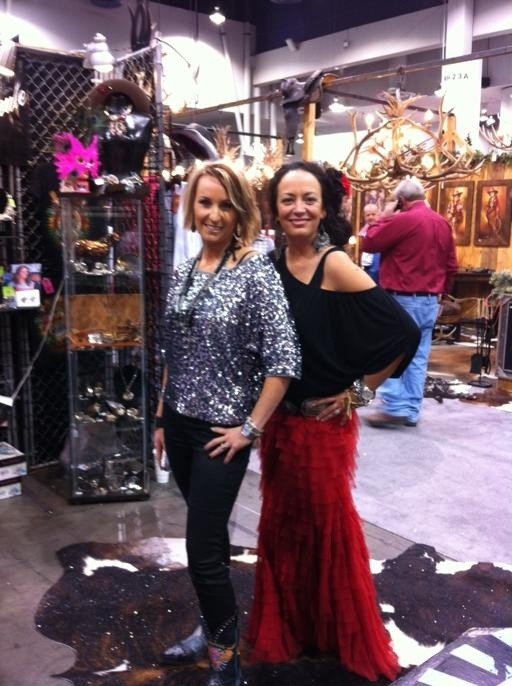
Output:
[119,363,139,400]
[178,237,240,339]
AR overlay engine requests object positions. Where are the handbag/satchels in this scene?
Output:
[104,106,155,141]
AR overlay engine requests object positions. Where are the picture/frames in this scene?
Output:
[475,179,511,246]
[439,181,473,246]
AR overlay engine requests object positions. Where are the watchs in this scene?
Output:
[149,414,167,429]
[351,377,376,408]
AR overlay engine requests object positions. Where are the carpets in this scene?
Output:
[33,534,511,685]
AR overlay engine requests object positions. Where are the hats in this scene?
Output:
[87,78,150,111]
[164,122,218,160]
[452,190,463,196]
[304,70,339,103]
[280,77,307,105]
[487,186,498,194]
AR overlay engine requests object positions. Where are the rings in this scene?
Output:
[220,441,228,451]
[334,408,341,415]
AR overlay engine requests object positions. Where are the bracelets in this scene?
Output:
[240,415,264,440]
[343,388,362,418]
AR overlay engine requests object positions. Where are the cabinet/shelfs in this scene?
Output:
[38,179,151,505]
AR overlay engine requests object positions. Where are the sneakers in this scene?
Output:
[367,412,407,425]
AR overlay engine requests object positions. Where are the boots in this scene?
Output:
[200,607,244,685]
[159,623,207,664]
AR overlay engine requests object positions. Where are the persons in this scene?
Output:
[357,175,463,431]
[484,185,502,238]
[444,188,464,237]
[239,164,419,682]
[12,266,40,291]
[361,202,381,286]
[145,161,304,684]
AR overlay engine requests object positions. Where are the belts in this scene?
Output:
[386,288,438,296]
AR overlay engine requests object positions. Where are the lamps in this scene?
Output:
[328,96,348,116]
[478,96,512,162]
[296,124,307,146]
[342,75,485,201]
[82,31,114,74]
[208,4,225,27]
